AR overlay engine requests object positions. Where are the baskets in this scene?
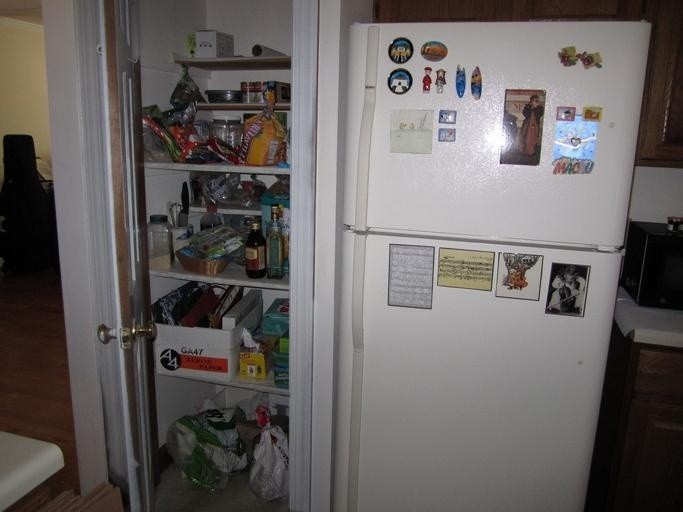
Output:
[175,251,228,276]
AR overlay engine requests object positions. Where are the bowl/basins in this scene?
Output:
[204,89,239,104]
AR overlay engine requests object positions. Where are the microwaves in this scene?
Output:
[619,220,683,311]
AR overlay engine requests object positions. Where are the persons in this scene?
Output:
[547,265,586,316]
[435,68,447,94]
[422,67,433,94]
[507,262,530,290]
[519,94,544,158]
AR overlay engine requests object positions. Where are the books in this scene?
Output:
[150,279,263,331]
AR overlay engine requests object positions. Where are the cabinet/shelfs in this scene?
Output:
[585,317,682,511]
[373,0,643,164]
[139,46,299,401]
[634,0,679,166]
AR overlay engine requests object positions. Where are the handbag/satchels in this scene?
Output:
[153,282,243,327]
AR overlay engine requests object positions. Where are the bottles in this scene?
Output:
[147,212,174,273]
[243,205,287,279]
[239,81,268,105]
[251,45,287,57]
[208,114,244,149]
[198,204,221,230]
[665,216,682,234]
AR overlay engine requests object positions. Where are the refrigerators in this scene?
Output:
[329,18,651,512]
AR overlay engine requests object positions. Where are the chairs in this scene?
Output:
[1,134,59,279]
